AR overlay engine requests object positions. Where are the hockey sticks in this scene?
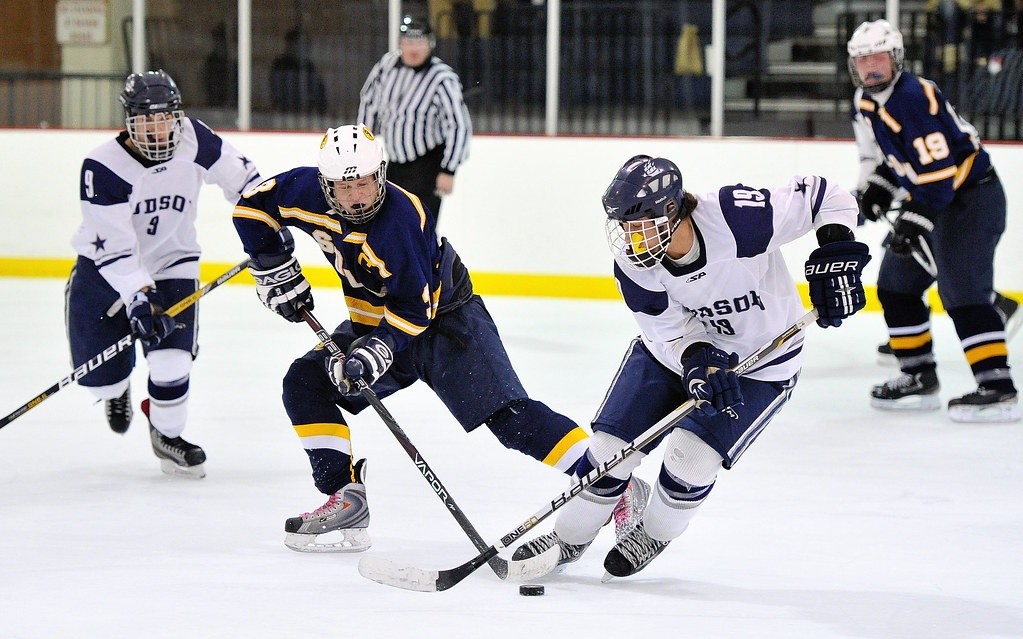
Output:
[0,258,252,430]
[850,190,938,278]
[357,307,820,594]
[292,301,561,582]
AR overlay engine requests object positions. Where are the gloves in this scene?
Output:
[863,163,901,221]
[325,330,396,396]
[804,242,873,328]
[126,289,176,347]
[253,248,314,323]
[884,200,937,260]
[679,341,745,418]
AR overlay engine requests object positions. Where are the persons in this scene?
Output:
[65,74,294,478]
[233,124,648,553]
[914,0,1023,98]
[203,23,239,111]
[848,18,1023,421]
[512,154,871,582]
[357,20,471,224]
[271,32,327,116]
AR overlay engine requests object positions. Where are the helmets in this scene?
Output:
[318,124,389,224]
[601,156,686,271]
[119,69,184,162]
[847,19,904,93]
[401,15,434,45]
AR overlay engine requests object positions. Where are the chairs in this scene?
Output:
[471,0,815,106]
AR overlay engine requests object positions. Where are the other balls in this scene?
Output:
[519,585,545,597]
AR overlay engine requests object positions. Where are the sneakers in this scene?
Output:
[141,398,207,480]
[286,458,370,554]
[614,475,653,542]
[103,382,133,434]
[947,378,1023,423]
[601,521,672,584]
[987,289,1023,340]
[870,363,940,412]
[512,514,612,578]
[875,304,931,362]
